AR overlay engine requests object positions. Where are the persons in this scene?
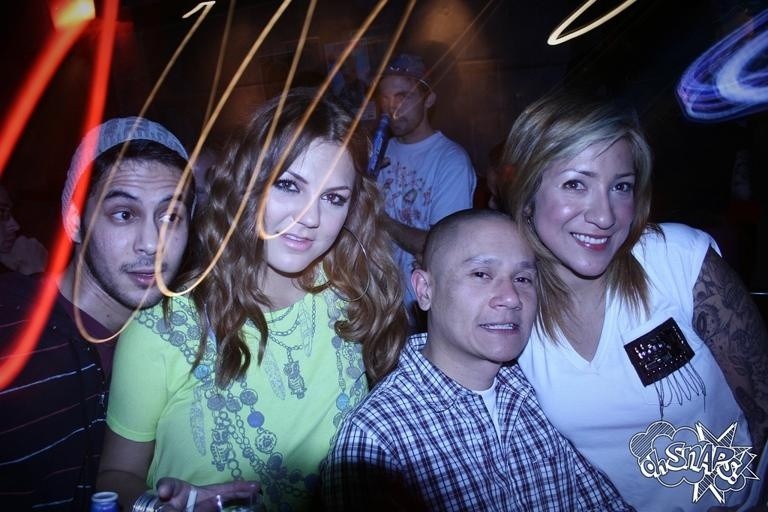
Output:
[0,54,768,512]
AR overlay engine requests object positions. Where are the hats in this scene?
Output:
[62,116,198,244]
[365,54,434,101]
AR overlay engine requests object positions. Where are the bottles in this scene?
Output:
[366,115,393,176]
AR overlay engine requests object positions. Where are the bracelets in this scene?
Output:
[132,493,159,512]
